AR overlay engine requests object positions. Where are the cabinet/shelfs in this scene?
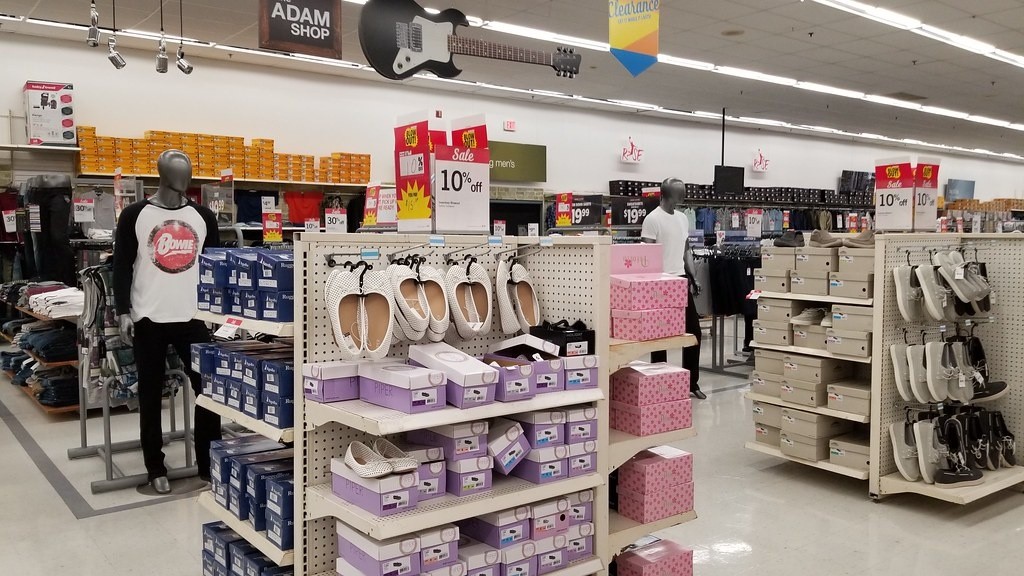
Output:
[0,299,169,415]
[745,233,1024,506]
[67,171,397,235]
[189,235,698,576]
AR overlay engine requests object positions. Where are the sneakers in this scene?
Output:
[821,308,833,328]
[809,230,843,248]
[789,308,826,326]
[888,250,1019,485]
[843,230,875,248]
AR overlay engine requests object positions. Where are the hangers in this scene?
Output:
[690,242,756,260]
[4,182,19,195]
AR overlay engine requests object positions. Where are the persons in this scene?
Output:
[23,174,77,281]
[112,147,223,494]
[641,177,707,399]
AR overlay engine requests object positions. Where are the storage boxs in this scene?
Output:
[300,341,599,576]
[751,246,875,469]
[213,532,243,569]
[209,435,294,551]
[228,570,237,576]
[243,552,277,576]
[195,246,295,322]
[227,539,259,576]
[202,521,231,557]
[609,446,694,523]
[202,550,215,576]
[609,361,693,436]
[261,565,294,576]
[190,338,294,429]
[213,560,229,576]
[73,125,371,185]
[609,169,876,208]
[608,535,694,576]
[23,80,78,146]
[942,198,1024,222]
[607,243,690,342]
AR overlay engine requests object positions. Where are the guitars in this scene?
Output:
[357,0,581,81]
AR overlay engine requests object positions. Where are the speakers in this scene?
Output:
[714,166,744,195]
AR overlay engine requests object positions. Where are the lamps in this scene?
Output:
[107,0,126,69]
[86,0,101,47]
[155,0,168,74]
[176,0,193,75]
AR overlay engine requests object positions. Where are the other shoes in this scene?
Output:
[372,437,418,474]
[324,264,450,360]
[774,230,805,247]
[538,318,587,334]
[468,263,493,336]
[345,440,393,479]
[512,263,540,334]
[494,260,521,335]
[446,265,480,340]
